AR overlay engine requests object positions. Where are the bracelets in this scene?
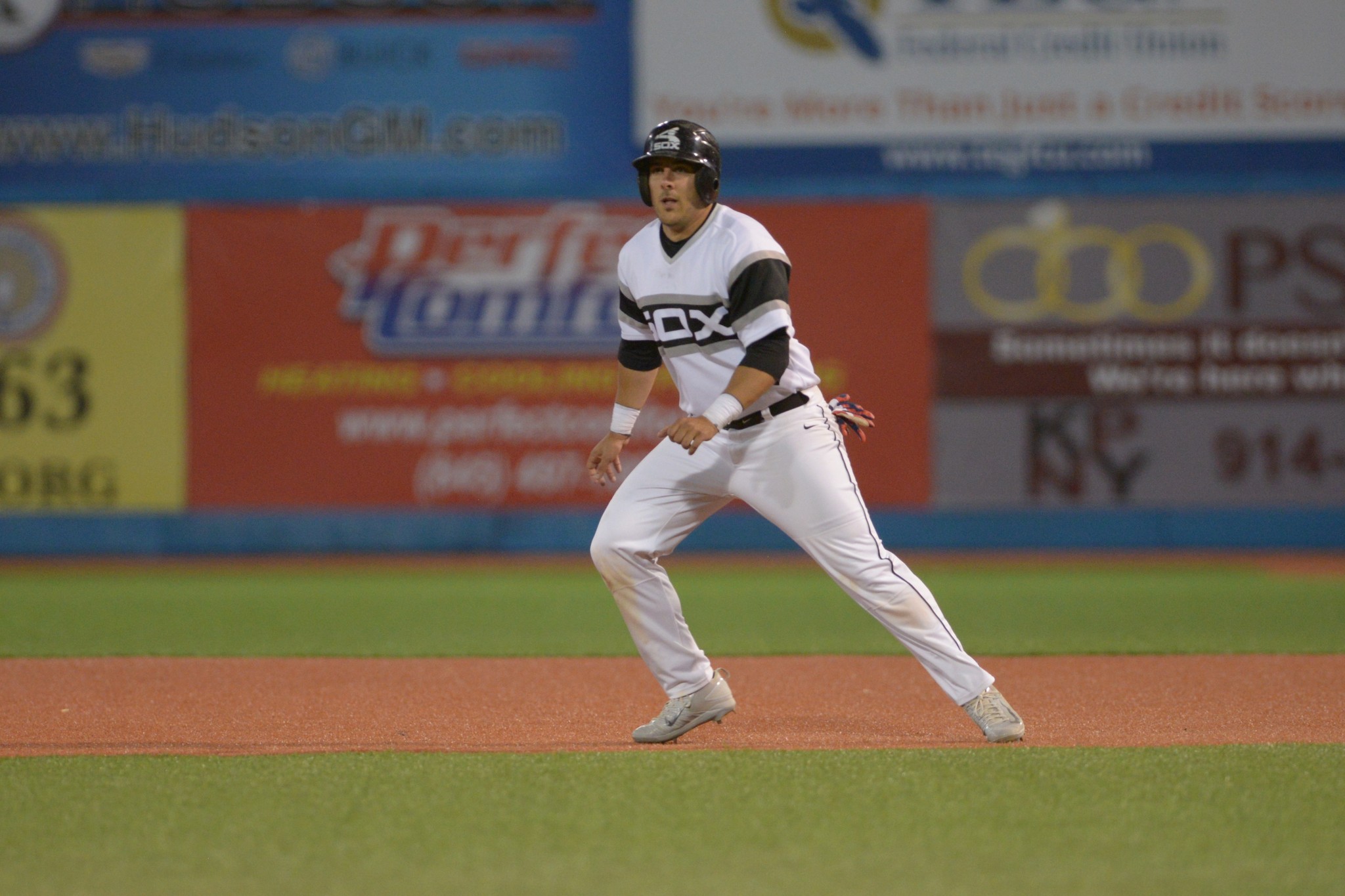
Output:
[609,402,641,435]
[702,393,744,433]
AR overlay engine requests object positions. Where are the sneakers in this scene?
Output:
[965,684,1026,742]
[631,667,736,744]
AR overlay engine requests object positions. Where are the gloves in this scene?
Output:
[829,393,875,441]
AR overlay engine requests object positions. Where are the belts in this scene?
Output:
[722,392,809,430]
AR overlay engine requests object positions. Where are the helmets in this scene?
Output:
[633,120,721,205]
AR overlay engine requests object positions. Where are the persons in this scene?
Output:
[586,119,1025,744]
